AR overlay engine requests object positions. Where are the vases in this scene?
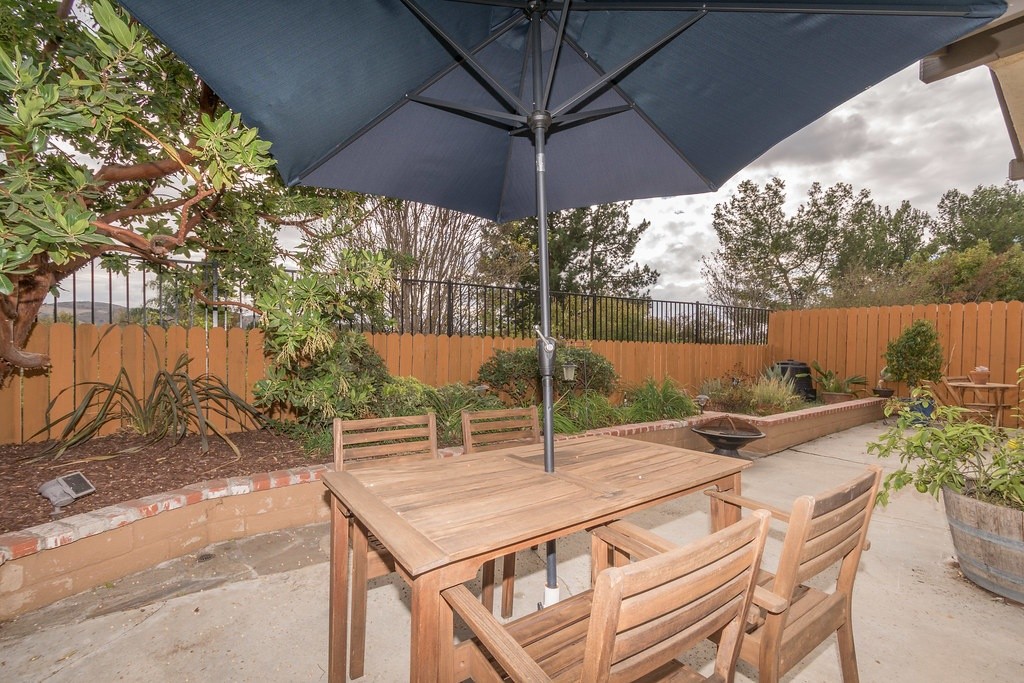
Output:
[968,370,992,385]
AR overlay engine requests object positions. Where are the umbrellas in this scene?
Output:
[108,0,1010,226]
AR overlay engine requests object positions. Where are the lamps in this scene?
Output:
[696,390,710,415]
[560,351,578,385]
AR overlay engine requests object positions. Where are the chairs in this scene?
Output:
[918,378,990,428]
[605,465,884,683]
[938,374,1013,416]
[461,404,541,619]
[438,508,772,683]
[332,411,452,680]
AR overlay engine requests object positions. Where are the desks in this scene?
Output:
[946,382,1018,432]
[321,435,753,683]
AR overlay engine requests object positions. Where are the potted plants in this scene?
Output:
[797,360,871,405]
[865,364,1024,606]
[872,366,897,398]
